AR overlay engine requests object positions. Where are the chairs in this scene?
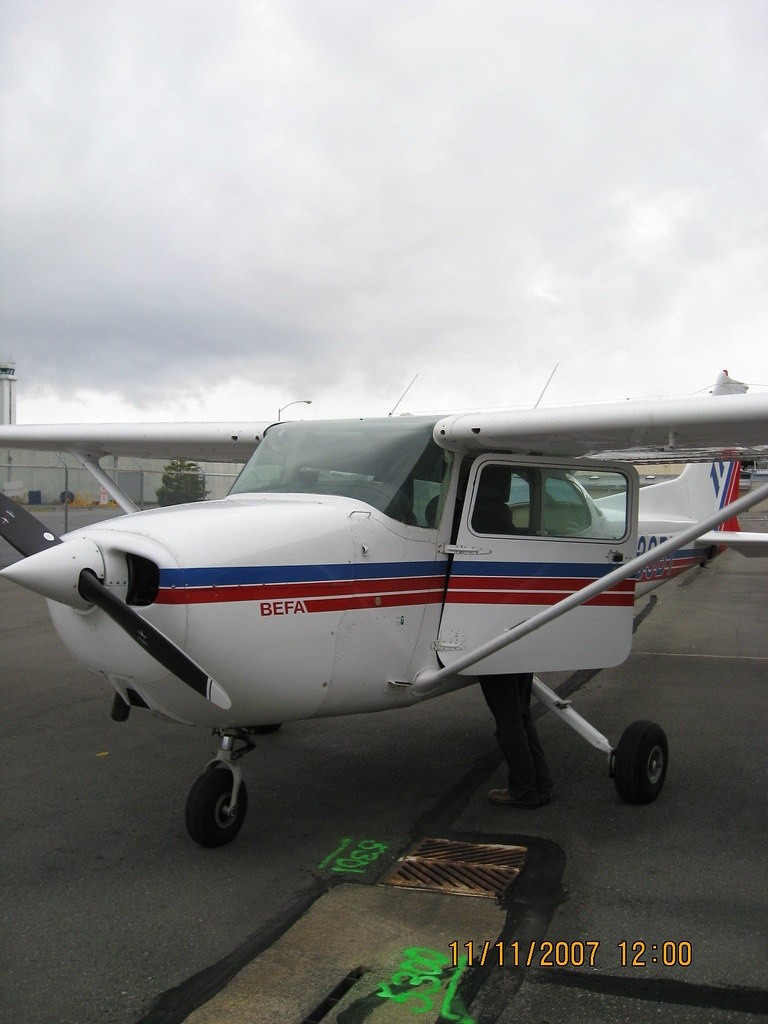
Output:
[425,463,514,535]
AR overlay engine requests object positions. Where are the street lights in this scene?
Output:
[277,398,313,423]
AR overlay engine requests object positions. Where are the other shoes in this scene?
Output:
[488,788,540,809]
[539,783,552,805]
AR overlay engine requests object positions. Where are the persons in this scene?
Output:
[424,479,553,809]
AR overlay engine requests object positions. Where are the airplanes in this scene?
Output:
[2,370,768,848]
